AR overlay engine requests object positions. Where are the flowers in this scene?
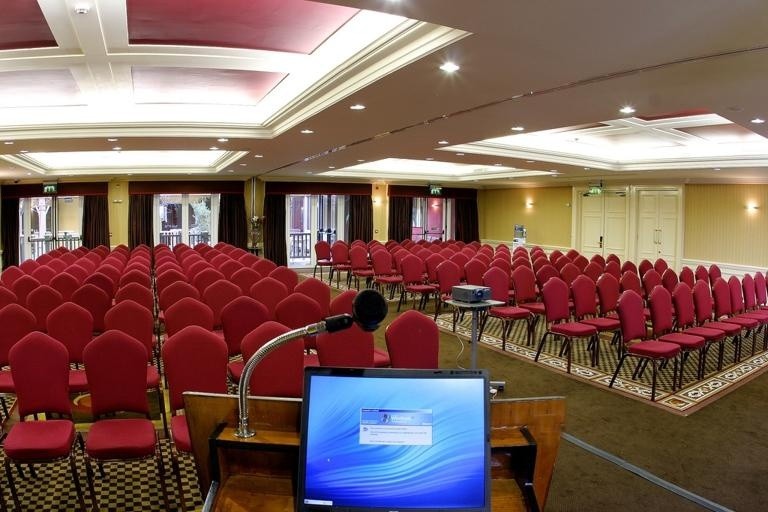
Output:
[249,215,265,228]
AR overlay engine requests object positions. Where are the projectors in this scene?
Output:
[452,285,491,303]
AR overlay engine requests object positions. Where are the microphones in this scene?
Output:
[234,289,388,434]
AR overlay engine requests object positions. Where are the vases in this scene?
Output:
[249,227,260,246]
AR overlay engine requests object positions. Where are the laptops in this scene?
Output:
[297,367,491,512]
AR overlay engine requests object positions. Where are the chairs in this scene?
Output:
[84,330,171,512]
[162,325,231,510]
[3,331,86,512]
